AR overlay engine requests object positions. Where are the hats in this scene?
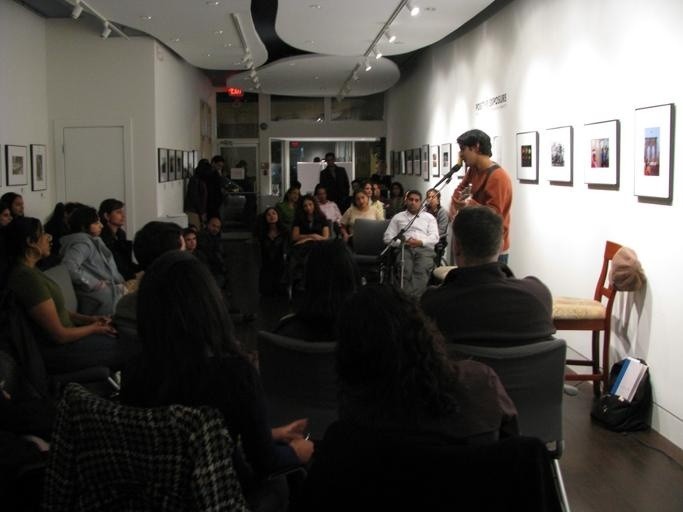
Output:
[609,247,647,292]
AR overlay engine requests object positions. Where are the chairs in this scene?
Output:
[1,212,574,511]
[541,241,638,403]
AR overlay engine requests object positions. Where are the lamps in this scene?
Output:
[334,0,420,103]
[71,0,130,42]
[230,11,263,95]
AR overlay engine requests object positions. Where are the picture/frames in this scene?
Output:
[544,124,572,183]
[4,144,28,186]
[582,119,617,185]
[514,130,538,181]
[29,143,48,192]
[156,146,199,183]
[630,102,671,198]
[386,142,456,184]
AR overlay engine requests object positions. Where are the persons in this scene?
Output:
[320,152,351,215]
[119,250,314,484]
[341,161,449,303]
[2,191,144,433]
[184,215,258,323]
[447,128,515,267]
[251,184,350,331]
[420,206,552,346]
[182,156,257,223]
[304,283,521,510]
[114,222,186,405]
[274,238,362,343]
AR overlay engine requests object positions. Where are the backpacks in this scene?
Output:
[589,358,652,433]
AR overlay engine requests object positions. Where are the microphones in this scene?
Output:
[433,164,461,189]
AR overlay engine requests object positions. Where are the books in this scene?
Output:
[608,357,648,405]
